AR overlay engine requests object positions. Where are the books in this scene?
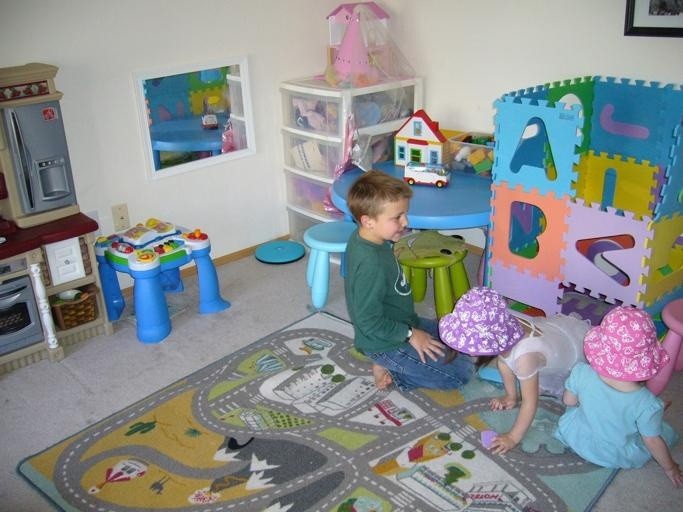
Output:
[40,236,92,288]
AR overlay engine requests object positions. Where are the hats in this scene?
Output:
[583,305,670,382]
[438,286,525,356]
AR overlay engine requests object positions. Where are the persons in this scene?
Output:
[342,170,474,392]
[552,305,682,491]
[437,284,592,456]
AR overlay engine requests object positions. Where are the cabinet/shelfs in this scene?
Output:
[278,75,424,266]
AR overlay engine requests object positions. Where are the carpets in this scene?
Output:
[15,310,620,512]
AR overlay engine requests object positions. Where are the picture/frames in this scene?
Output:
[131,55,257,184]
[624,0,683,37]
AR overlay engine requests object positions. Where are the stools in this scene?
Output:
[392,229,470,321]
[303,220,358,309]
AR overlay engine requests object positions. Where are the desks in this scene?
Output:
[331,159,493,279]
